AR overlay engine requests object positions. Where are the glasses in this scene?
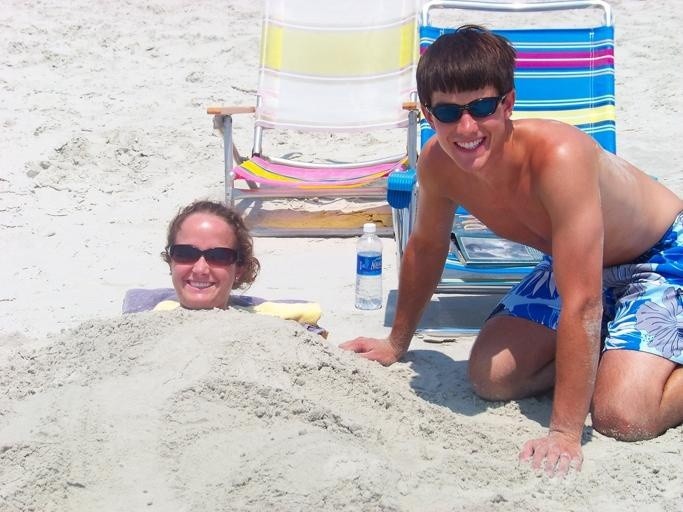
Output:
[169,244,243,268]
[423,95,505,123]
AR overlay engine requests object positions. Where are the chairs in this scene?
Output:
[386,4,615,296]
[208,4,418,275]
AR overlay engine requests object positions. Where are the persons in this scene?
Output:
[337,23,683,480]
[163,200,258,310]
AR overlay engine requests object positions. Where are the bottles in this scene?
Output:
[356,222,386,311]
